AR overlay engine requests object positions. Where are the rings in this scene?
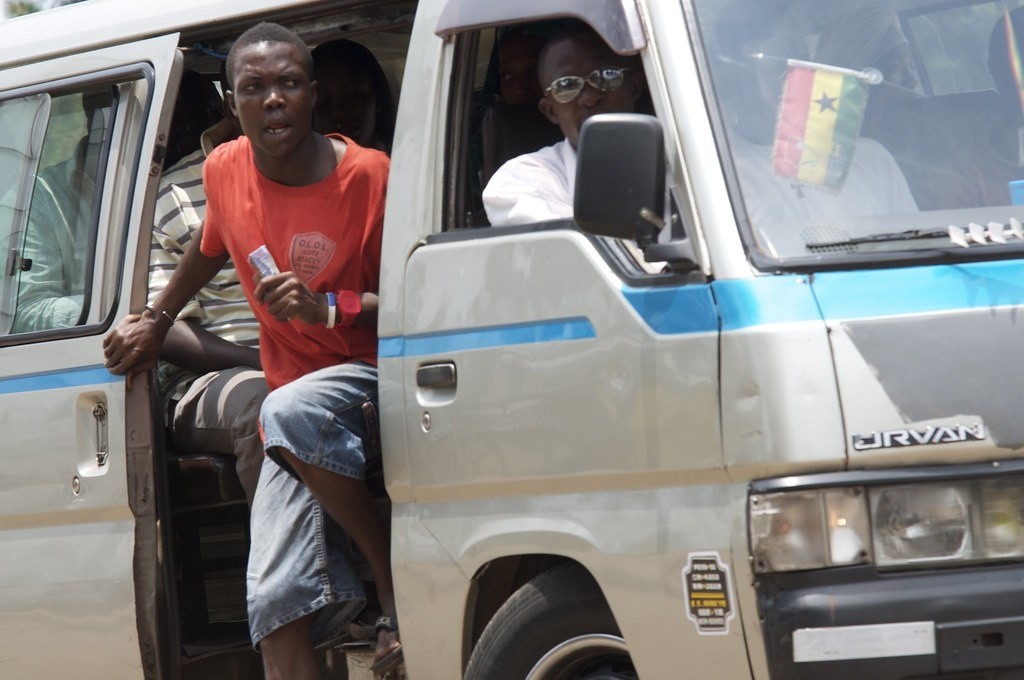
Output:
[132,347,143,352]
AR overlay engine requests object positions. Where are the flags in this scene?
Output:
[771,58,871,191]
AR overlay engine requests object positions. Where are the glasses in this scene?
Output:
[545,65,632,104]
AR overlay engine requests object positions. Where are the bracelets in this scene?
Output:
[324,292,336,329]
[144,304,175,327]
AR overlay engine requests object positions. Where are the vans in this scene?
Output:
[0,0,1024,680]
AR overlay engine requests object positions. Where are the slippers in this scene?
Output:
[370,615,403,677]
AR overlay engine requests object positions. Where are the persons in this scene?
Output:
[99,23,405,680]
[12,78,118,335]
[465,27,564,228]
[141,62,280,520]
[157,68,228,176]
[302,37,399,156]
[483,26,641,227]
[713,0,1024,224]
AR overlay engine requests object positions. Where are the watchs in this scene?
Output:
[336,289,363,330]
